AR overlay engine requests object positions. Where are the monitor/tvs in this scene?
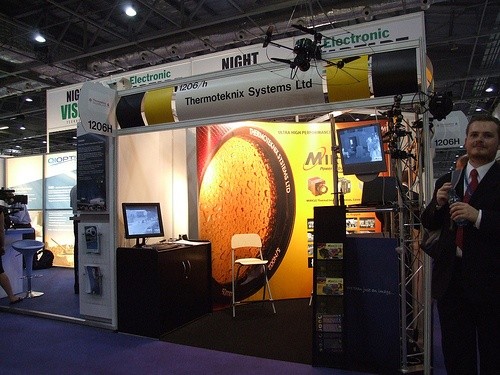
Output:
[336,123,386,175]
[122,203,164,249]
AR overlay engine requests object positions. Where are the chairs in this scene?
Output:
[231,234,277,319]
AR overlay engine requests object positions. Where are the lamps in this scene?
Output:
[6,0,435,97]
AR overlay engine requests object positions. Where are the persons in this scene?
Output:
[0,187,35,305]
[421,113,500,375]
[70,185,80,295]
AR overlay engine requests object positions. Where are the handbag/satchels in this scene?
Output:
[36,250,54,268]
[420,171,462,258]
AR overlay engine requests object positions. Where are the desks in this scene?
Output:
[118,239,213,340]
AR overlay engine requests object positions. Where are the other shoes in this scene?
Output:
[9,295,24,303]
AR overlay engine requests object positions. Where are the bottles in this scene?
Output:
[447,189,468,228]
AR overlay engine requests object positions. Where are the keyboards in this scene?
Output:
[154,243,180,251]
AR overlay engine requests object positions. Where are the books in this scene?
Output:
[317,243,343,259]
[85,225,100,254]
[83,265,103,296]
[316,277,344,295]
[317,312,343,354]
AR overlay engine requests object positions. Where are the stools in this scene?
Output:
[10,239,45,300]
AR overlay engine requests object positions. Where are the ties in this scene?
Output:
[456,169,480,251]
